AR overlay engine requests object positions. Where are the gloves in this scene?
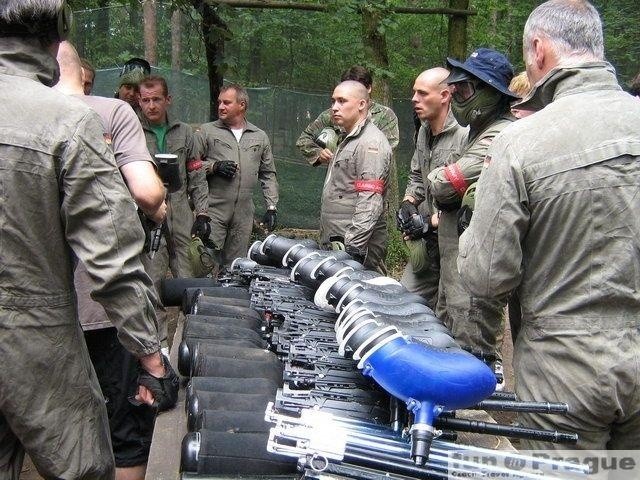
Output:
[191,214,212,247]
[212,160,239,181]
[456,204,472,237]
[126,354,180,416]
[261,209,278,234]
[397,200,433,241]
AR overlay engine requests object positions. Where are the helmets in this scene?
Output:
[402,236,445,274]
[118,57,151,90]
[189,237,217,278]
[438,66,510,128]
[314,125,341,160]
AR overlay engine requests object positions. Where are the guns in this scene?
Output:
[161,233,578,479]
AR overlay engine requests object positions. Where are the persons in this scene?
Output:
[3,1,640,480]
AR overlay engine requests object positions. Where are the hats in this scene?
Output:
[446,47,523,106]
